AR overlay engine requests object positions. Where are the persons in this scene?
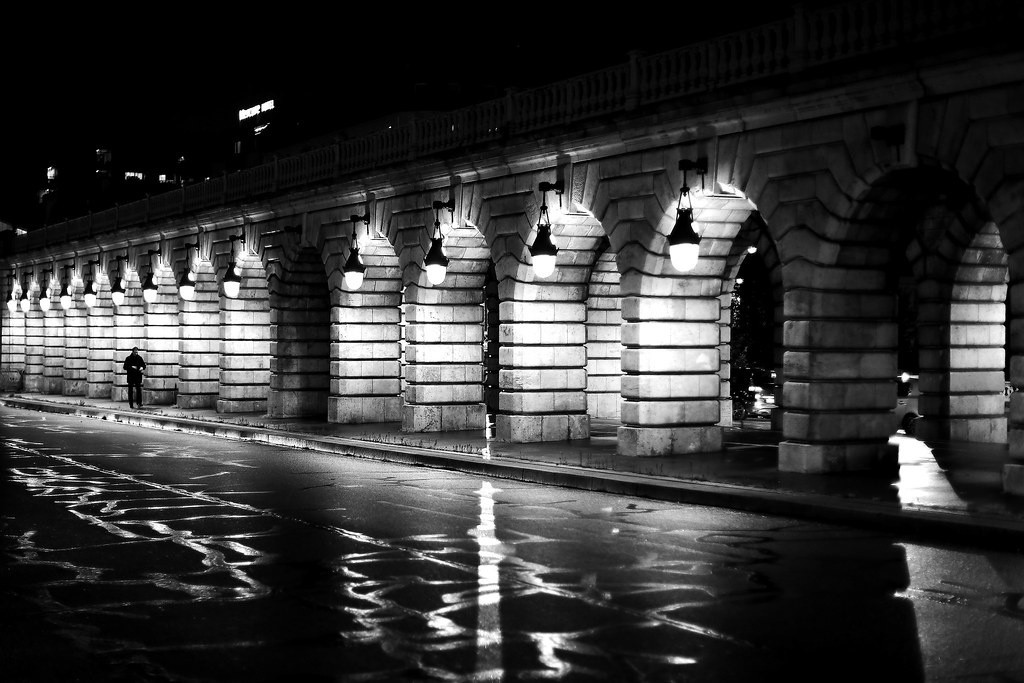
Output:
[123,347,146,408]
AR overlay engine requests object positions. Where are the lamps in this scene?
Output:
[5,155,708,313]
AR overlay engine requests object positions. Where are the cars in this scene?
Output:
[728,365,777,420]
[892,374,923,436]
